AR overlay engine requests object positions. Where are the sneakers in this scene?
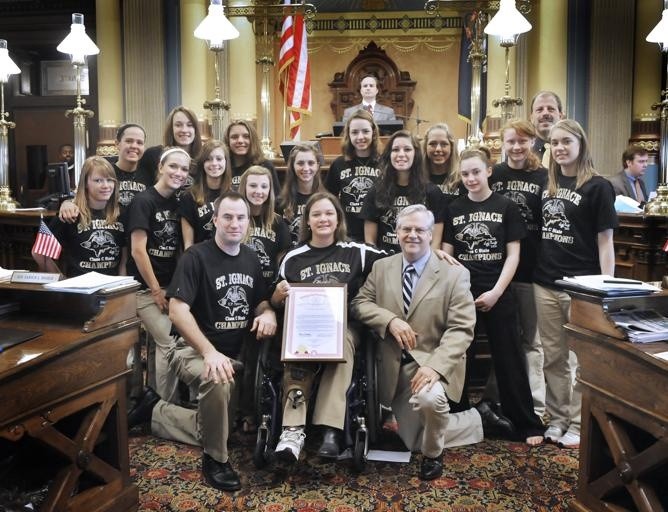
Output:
[472,397,581,449]
[274,427,306,461]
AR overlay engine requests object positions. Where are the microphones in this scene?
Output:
[363,104,369,110]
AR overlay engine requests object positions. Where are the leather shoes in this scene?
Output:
[127,386,161,433]
[421,449,443,480]
[315,426,339,457]
[202,451,241,491]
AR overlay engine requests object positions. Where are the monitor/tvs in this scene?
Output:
[47,162,71,197]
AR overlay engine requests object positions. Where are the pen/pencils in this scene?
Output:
[604,280,643,284]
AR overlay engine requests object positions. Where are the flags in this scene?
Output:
[278,0,313,143]
[457,11,489,132]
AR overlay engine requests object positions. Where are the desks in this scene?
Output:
[614,213,668,284]
[0,272,147,512]
[559,277,668,512]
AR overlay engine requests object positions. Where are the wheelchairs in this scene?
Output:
[253,319,373,470]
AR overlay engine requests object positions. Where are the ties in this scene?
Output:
[541,143,551,170]
[402,265,415,365]
[635,180,644,204]
